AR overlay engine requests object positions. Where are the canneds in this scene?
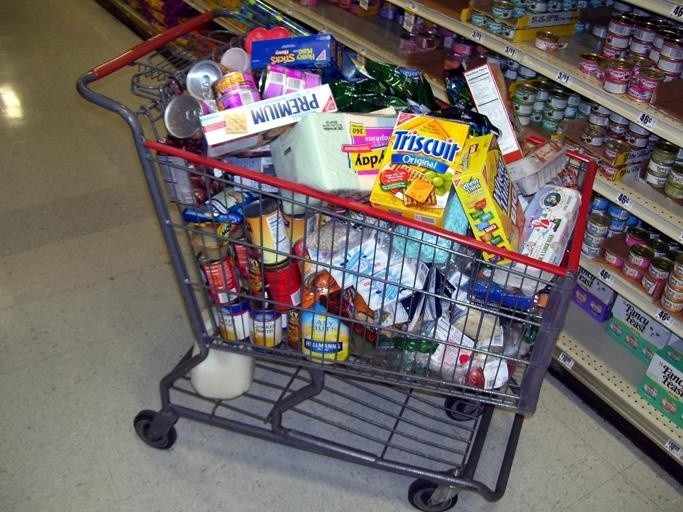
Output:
[379,1,683,315]
[153,46,350,366]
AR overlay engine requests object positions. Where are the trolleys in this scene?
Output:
[76,6,597,512]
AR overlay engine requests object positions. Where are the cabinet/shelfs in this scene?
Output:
[111,0,683,465]
